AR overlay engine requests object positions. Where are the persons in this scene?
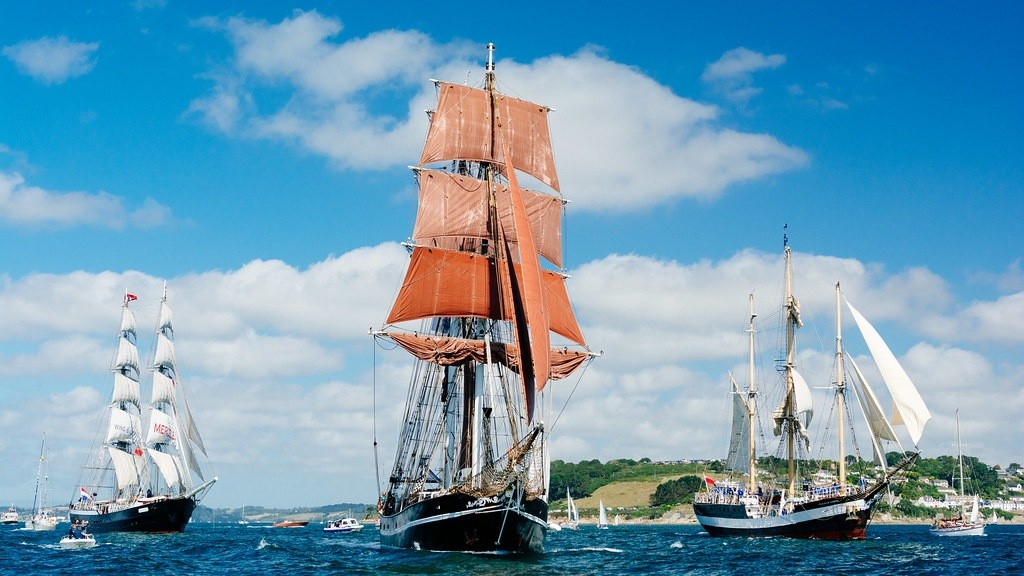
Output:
[147,488,152,497]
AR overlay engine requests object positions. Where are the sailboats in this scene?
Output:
[367,42,604,554]
[68,278,218,535]
[597,500,609,530]
[560,487,579,529]
[989,511,997,525]
[25,432,56,530]
[929,408,987,537]
[692,223,932,541]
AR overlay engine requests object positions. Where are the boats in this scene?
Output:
[0,508,19,525]
[546,521,561,532]
[274,520,309,528]
[60,524,96,550]
[238,520,249,525]
[247,522,276,529]
[323,519,364,533]
[374,518,381,530]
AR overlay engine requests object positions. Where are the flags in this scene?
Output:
[80,489,94,501]
[704,475,716,485]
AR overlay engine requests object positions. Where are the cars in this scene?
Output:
[978,501,991,506]
